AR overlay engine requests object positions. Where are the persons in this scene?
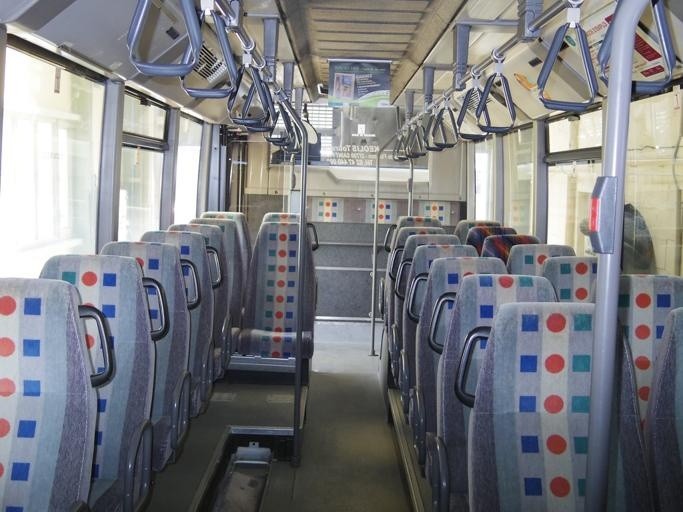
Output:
[580,203,660,276]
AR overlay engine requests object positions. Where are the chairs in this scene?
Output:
[0,230,223,512]
[167,208,320,380]
[381,216,682,511]
